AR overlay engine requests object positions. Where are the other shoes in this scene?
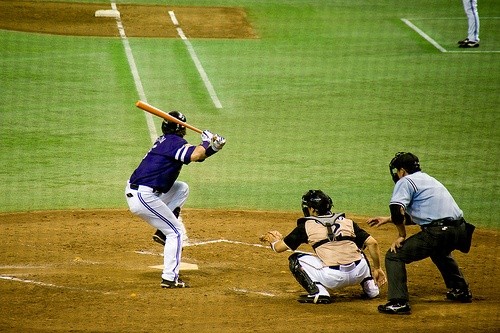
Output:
[371,294,380,300]
[459,41,479,48]
[458,38,469,44]
[297,295,329,303]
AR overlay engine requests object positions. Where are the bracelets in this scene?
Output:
[205,146,215,157]
[200,141,209,148]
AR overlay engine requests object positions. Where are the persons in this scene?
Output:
[259,191,387,304]
[366,153,473,315]
[126,111,226,288]
[458,0,481,48]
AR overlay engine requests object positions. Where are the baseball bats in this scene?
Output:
[135,101,216,140]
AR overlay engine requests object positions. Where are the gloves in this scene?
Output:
[201,130,213,144]
[211,134,226,151]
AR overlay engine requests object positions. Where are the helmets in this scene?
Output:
[162,111,186,135]
[395,151,421,174]
[307,189,331,215]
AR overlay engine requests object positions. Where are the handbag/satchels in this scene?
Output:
[456,223,475,253]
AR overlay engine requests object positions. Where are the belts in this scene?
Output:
[130,183,156,193]
[329,260,360,270]
[432,217,464,226]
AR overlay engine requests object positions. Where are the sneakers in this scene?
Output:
[378,302,411,314]
[153,229,183,251]
[446,290,473,302]
[160,278,188,287]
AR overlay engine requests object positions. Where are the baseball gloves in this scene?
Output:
[258,230,284,247]
